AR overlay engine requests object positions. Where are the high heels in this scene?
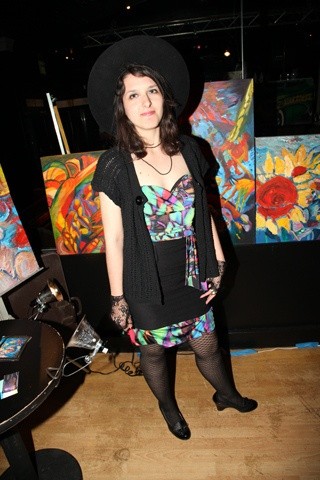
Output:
[158,411,193,442]
[211,392,259,414]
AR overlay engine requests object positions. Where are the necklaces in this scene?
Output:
[140,143,173,175]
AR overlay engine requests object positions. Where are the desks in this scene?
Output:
[0,319,84,480]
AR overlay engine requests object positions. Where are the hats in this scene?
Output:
[86,35,192,137]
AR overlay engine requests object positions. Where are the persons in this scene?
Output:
[92,65,258,440]
